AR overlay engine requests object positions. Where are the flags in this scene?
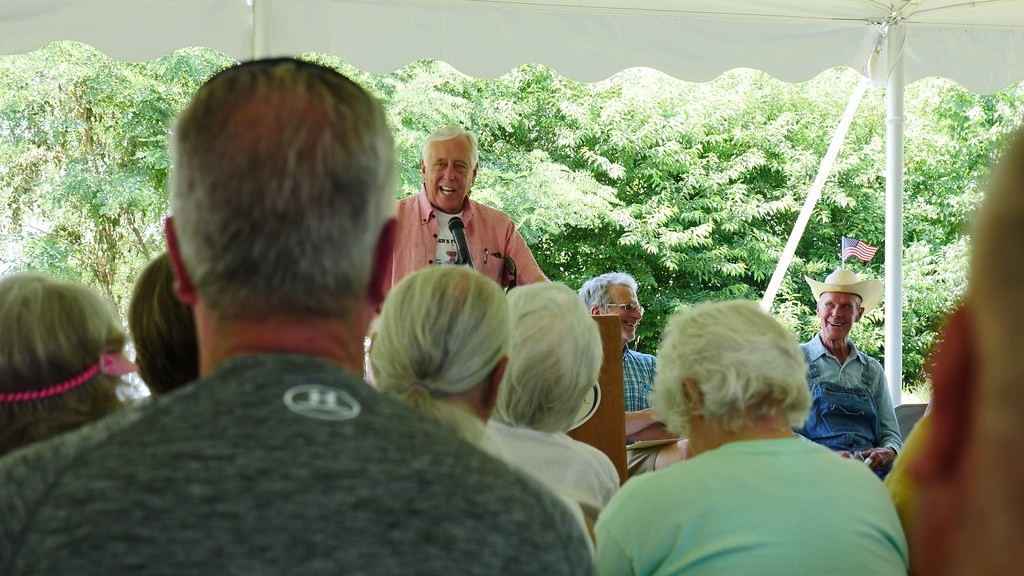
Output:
[843,237,878,261]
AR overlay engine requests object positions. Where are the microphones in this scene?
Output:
[448,217,473,268]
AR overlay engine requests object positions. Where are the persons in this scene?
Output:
[882,119,1024,575]
[0,272,139,457]
[476,281,622,551]
[367,124,553,340]
[590,302,912,575]
[370,263,595,549]
[127,252,199,399]
[578,272,693,479]
[796,269,904,484]
[0,58,593,576]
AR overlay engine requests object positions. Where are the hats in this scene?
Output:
[805,269,883,315]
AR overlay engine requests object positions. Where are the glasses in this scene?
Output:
[606,304,644,312]
[501,255,517,294]
